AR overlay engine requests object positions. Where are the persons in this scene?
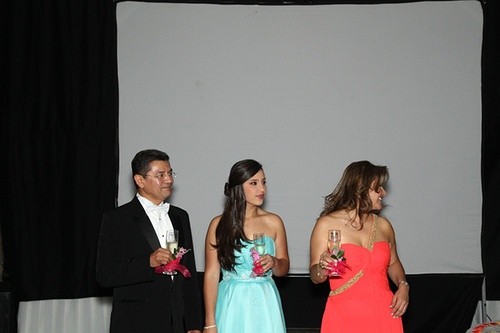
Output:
[310,160,409,333]
[97,149,202,333]
[203,159,290,333]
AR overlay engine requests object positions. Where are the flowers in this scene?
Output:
[322,246,354,280]
[155,247,191,278]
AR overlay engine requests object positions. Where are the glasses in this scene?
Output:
[143,172,177,180]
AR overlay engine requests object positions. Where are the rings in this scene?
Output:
[320,259,322,263]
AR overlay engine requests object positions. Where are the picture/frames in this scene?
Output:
[248,248,263,278]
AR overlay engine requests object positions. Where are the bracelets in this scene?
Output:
[204,325,216,328]
[398,281,408,286]
[316,264,327,280]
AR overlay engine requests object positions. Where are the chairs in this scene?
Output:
[18,298,111,333]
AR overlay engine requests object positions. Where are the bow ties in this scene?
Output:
[146,203,170,217]
